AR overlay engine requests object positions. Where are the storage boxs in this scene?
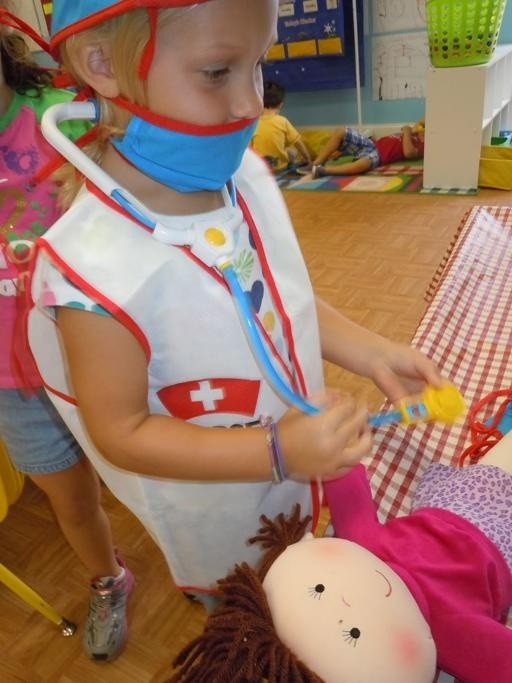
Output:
[478,145,511,192]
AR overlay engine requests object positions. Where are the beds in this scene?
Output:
[306,206,512,539]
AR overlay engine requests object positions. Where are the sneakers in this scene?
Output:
[85,562,134,662]
[296,161,325,179]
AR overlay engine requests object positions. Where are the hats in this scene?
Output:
[52,0,195,48]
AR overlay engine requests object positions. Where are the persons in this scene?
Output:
[171,427,512,683]
[295,117,425,180]
[248,82,311,174]
[0,33,198,663]
[21,0,442,616]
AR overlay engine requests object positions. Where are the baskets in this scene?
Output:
[429,0,504,68]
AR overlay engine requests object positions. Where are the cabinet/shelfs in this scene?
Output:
[422,44,512,189]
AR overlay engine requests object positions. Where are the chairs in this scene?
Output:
[1,440,78,637]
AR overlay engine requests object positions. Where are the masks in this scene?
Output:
[105,95,259,194]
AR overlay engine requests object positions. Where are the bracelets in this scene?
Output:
[264,426,281,483]
[272,423,285,480]
